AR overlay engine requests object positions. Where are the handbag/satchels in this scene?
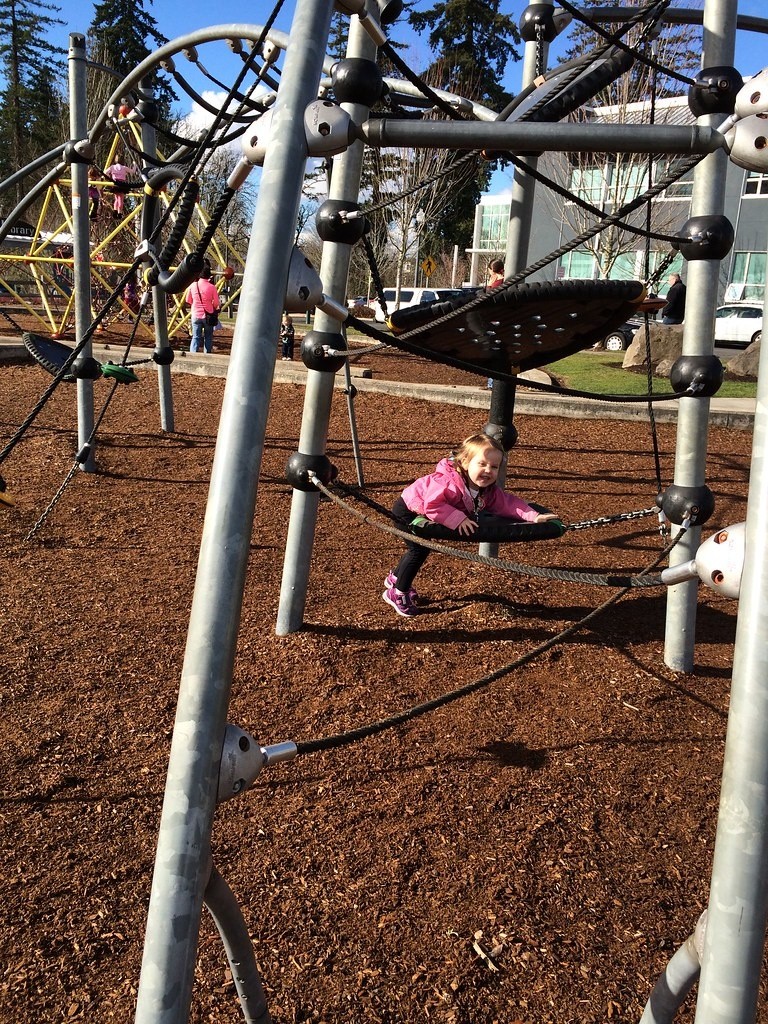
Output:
[213,320,221,331]
[204,310,218,326]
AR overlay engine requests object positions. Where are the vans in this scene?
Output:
[374,286,465,325]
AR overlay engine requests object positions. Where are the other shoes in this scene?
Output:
[282,357,290,361]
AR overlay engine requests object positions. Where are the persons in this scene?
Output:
[662,272,686,325]
[88,168,101,222]
[478,259,505,390]
[279,316,294,361]
[382,434,564,617]
[104,154,136,219]
[186,269,218,353]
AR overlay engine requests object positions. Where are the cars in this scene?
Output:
[347,295,374,309]
[712,304,764,348]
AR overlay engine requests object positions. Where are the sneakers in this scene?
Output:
[384,569,420,603]
[382,587,418,617]
